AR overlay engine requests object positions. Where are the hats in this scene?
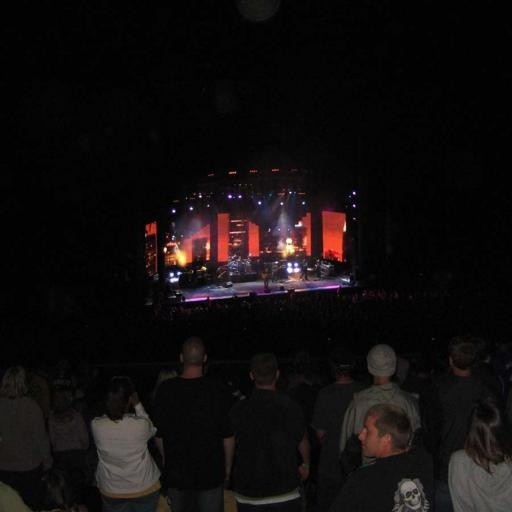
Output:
[251,351,283,385]
[366,344,398,378]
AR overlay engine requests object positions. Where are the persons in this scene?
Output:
[1,257,511,512]
